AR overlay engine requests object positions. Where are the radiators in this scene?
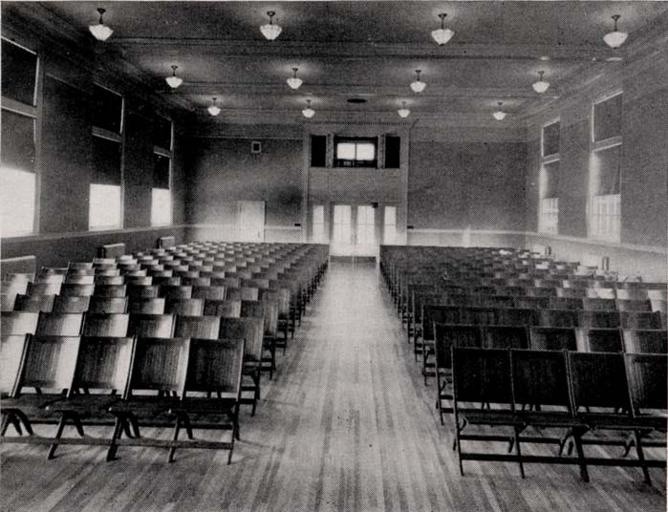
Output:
[159,236,175,250]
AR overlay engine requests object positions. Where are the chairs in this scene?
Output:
[379,245,668,485]
[0,241,329,464]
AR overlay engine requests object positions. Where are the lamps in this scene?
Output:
[208,98,220,116]
[261,10,282,41]
[431,12,456,47]
[494,100,508,123]
[88,8,114,42]
[411,69,427,95]
[166,65,183,89]
[398,101,411,118]
[301,99,316,119]
[286,69,303,90]
[532,70,550,95]
[603,14,629,49]
